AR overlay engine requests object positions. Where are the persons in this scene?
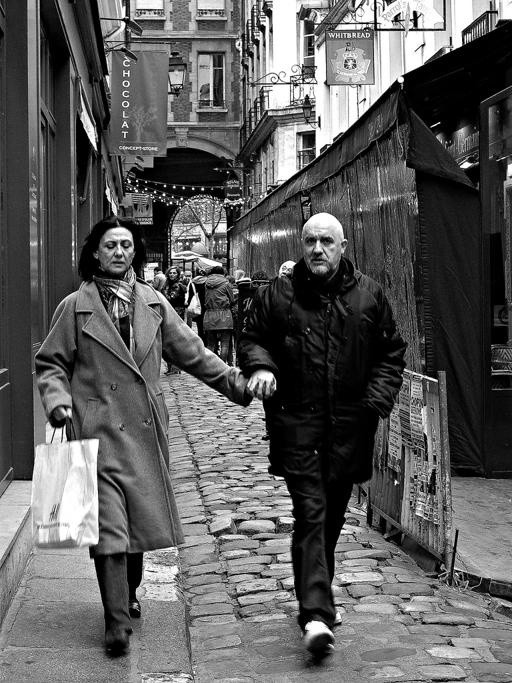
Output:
[146,259,267,375]
[236,211,407,656]
[32,218,278,658]
[279,259,298,277]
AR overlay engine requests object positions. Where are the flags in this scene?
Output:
[110,47,166,157]
[130,191,154,225]
[323,27,375,85]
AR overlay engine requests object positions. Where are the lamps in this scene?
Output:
[168,51,187,96]
[100,16,143,62]
[301,94,321,129]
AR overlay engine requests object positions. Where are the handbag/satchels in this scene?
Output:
[31,439,100,549]
[187,293,202,319]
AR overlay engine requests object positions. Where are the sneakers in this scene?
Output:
[127,597,141,617]
[104,629,129,652]
[303,620,335,656]
[334,606,341,625]
